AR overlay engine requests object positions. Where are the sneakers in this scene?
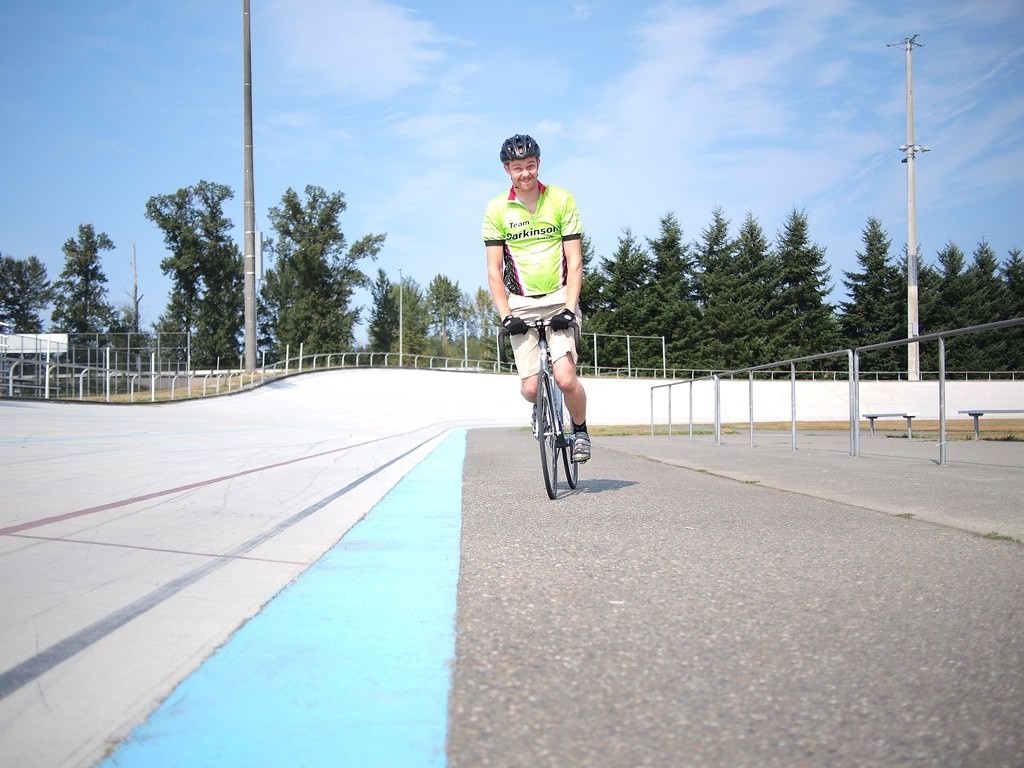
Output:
[572,432,591,461]
[531,397,549,441]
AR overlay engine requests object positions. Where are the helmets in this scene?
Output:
[500,134,541,164]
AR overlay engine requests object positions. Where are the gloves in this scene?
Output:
[502,315,528,335]
[551,309,575,332]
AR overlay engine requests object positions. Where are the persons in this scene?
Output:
[483,133,591,462]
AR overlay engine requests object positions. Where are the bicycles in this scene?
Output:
[498,317,588,500]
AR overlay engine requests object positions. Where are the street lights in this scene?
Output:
[885,33,931,381]
[398,268,403,368]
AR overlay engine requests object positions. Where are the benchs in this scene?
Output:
[958,410,1024,442]
[863,413,916,439]
